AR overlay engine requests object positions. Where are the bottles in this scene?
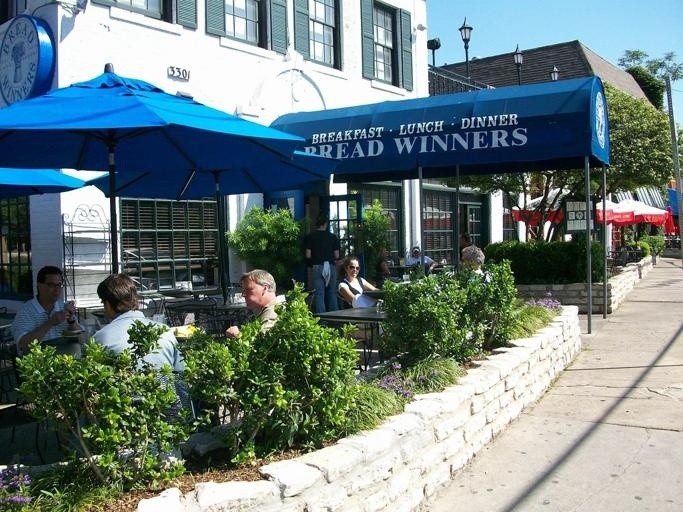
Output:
[228,294,237,304]
[375,299,384,313]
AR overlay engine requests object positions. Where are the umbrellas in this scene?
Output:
[617,199,669,225]
[0,169,86,197]
[511,187,636,227]
[1,62,305,274]
[665,206,675,245]
[85,150,340,304]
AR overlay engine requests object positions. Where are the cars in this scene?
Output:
[422,205,451,219]
[121,246,175,269]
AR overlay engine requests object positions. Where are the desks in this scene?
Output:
[390,262,456,280]
[0,282,390,465]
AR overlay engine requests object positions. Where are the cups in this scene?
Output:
[399,258,405,266]
[152,313,164,324]
[402,275,409,283]
[442,259,445,264]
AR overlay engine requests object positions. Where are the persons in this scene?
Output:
[193,269,279,425]
[11,267,85,358]
[90,272,195,422]
[306,214,485,339]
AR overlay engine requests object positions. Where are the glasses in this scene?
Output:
[350,266,360,271]
[45,282,64,289]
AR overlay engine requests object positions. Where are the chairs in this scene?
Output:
[608,246,642,275]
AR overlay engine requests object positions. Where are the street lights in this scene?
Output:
[511,40,524,90]
[457,17,473,84]
[548,63,560,87]
